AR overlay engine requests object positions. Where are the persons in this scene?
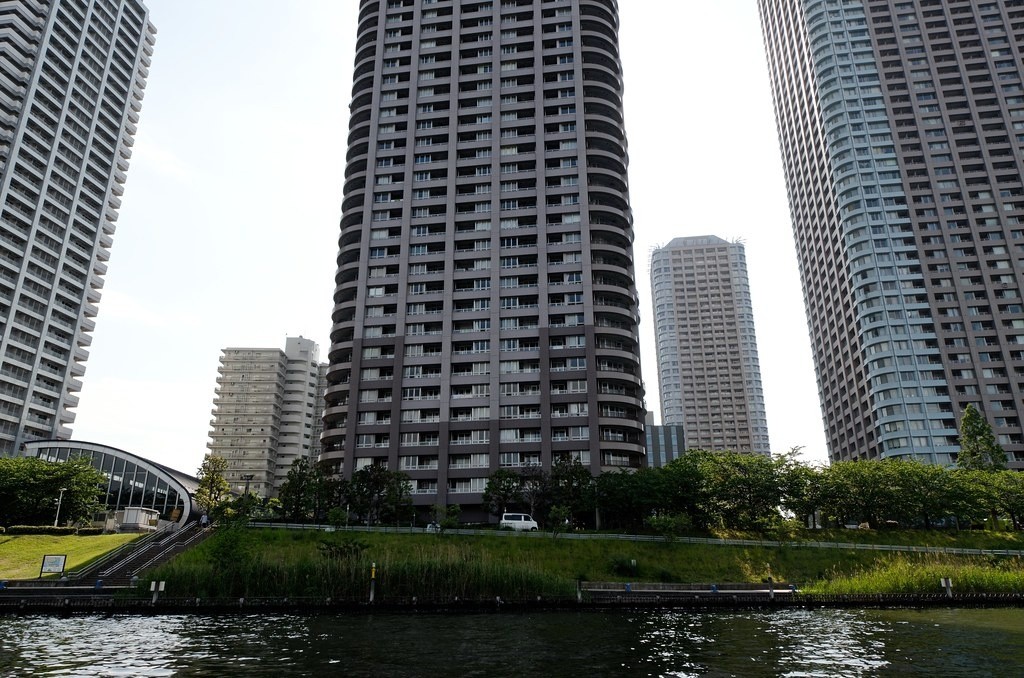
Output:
[66,516,82,534]
[199,511,207,528]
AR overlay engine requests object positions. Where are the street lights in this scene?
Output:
[504,507,507,513]
[54,487,68,526]
[499,513,539,531]
[346,503,351,529]
[413,513,416,526]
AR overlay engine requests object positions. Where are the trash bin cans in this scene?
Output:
[789,584,795,591]
[95,579,103,589]
[624,583,631,592]
[0,581,8,591]
[711,584,716,594]
